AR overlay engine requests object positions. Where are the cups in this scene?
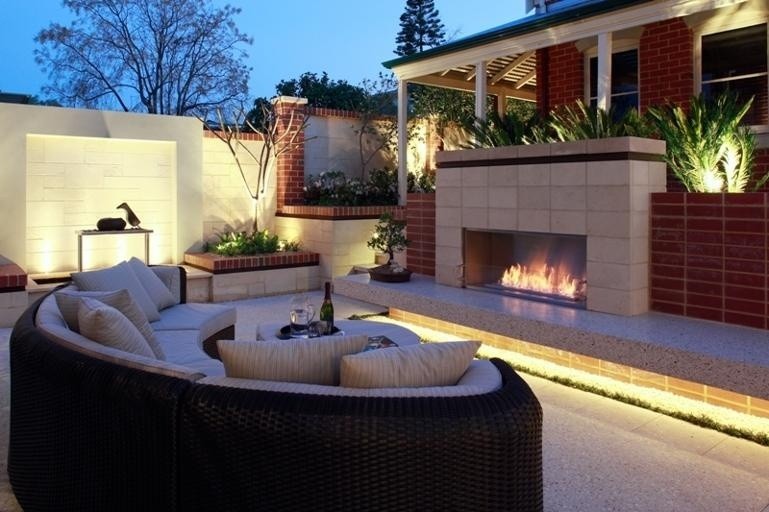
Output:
[288,293,321,339]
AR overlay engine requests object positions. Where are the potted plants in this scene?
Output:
[366,211,414,284]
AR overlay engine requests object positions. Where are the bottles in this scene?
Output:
[319,282,333,336]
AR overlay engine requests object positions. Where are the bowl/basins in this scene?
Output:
[97,218,126,231]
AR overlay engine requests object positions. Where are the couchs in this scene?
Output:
[6,264,547,512]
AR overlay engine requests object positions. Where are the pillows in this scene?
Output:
[49,256,180,363]
[213,335,482,386]
